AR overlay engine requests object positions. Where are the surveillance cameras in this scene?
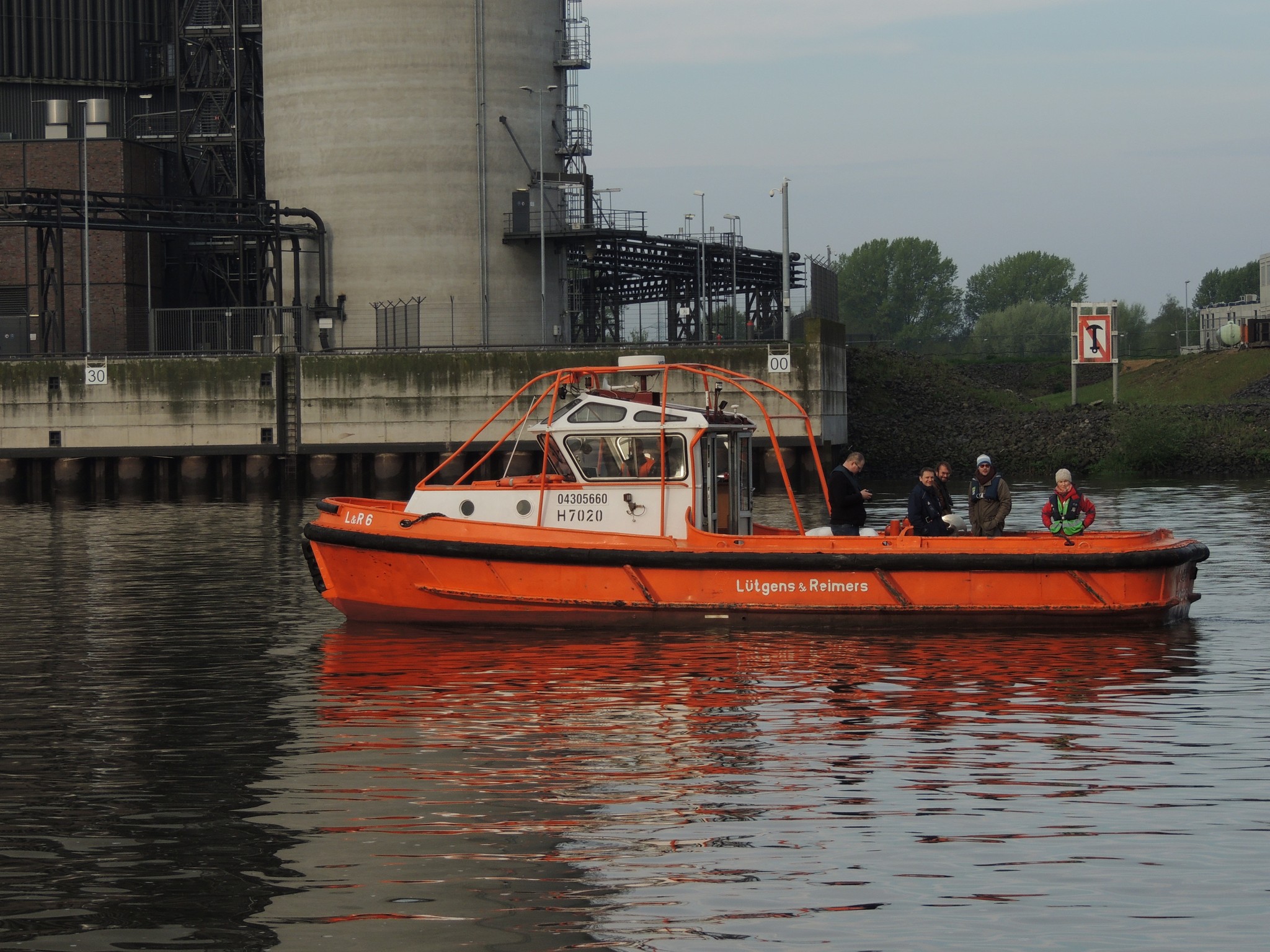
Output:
[769,191,775,197]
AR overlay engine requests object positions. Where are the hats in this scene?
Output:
[1055,468,1072,484]
[977,454,992,468]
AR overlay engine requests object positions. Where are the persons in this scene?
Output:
[619,438,657,478]
[648,437,681,477]
[828,452,873,536]
[931,461,953,517]
[1042,468,1096,544]
[968,454,1012,536]
[907,467,955,537]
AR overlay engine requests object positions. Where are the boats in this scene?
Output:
[303,358,1210,629]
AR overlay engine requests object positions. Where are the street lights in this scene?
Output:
[723,213,738,345]
[693,190,705,345]
[1185,279,1191,346]
[518,85,558,349]
[43,97,112,358]
[769,182,791,342]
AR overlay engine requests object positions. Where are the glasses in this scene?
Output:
[942,472,950,476]
[854,462,862,470]
[980,464,990,468]
